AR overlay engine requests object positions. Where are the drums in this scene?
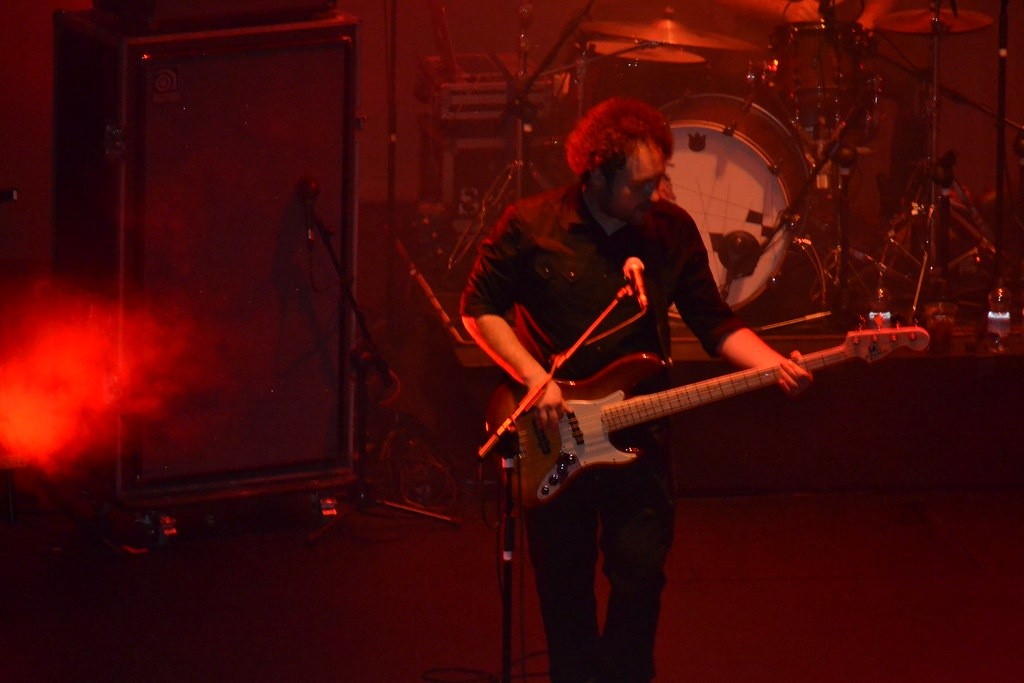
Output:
[779,22,861,109]
[648,95,811,313]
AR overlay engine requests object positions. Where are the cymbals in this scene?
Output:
[575,39,705,64]
[875,6,992,37]
[578,6,758,53]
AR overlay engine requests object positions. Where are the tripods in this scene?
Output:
[303,216,465,548]
[803,0,1024,336]
[436,0,550,291]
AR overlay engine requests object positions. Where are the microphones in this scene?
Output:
[623,257,647,312]
[299,178,321,243]
[722,235,751,300]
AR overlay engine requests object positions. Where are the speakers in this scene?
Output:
[48,1,366,519]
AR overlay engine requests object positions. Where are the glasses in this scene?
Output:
[604,163,662,198]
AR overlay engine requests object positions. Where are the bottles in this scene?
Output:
[869,289,890,327]
[988,279,1011,337]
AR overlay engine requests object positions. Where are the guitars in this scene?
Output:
[485,320,929,506]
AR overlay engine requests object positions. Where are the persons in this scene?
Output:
[461,98,814,683]
